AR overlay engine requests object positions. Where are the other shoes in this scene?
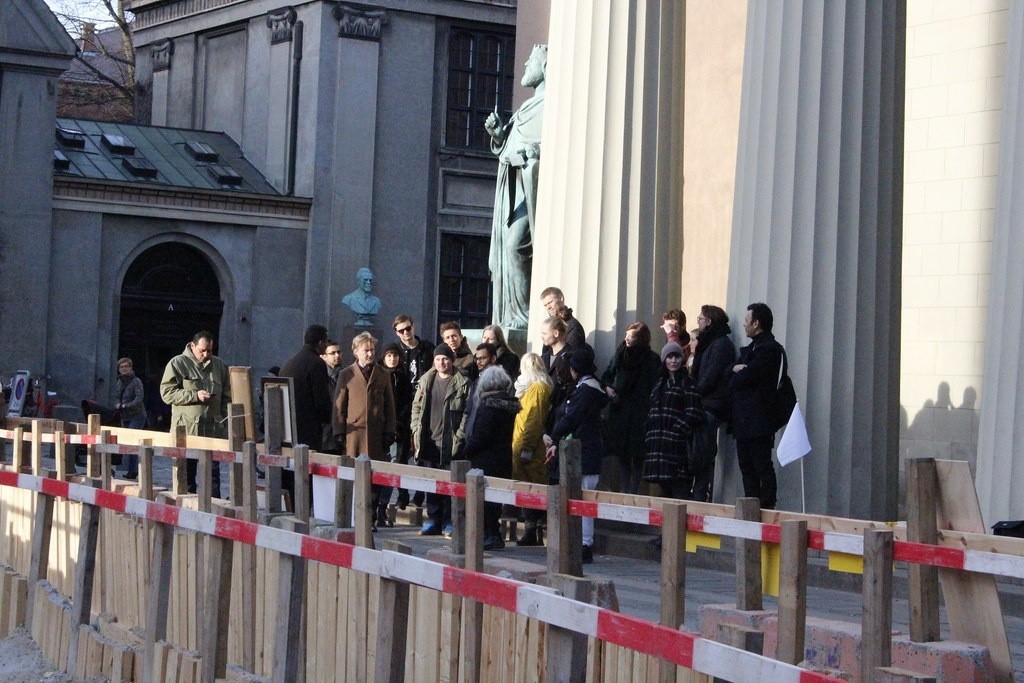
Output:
[418,523,443,535]
[123,471,139,479]
[445,522,453,539]
[484,537,506,551]
[582,544,594,565]
[648,534,663,546]
[515,527,539,546]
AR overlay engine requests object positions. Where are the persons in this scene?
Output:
[160,331,232,500]
[378,286,798,565]
[112,357,151,480]
[485,44,547,327]
[255,324,341,512]
[332,332,398,531]
[342,268,381,326]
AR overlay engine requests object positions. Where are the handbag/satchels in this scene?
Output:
[774,373,797,434]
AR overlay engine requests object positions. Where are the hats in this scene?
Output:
[380,341,400,359]
[433,343,455,363]
[661,340,685,362]
[567,343,598,374]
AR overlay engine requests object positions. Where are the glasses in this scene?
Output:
[394,323,412,336]
[473,355,490,362]
[326,350,341,356]
[697,315,708,322]
[119,365,131,369]
